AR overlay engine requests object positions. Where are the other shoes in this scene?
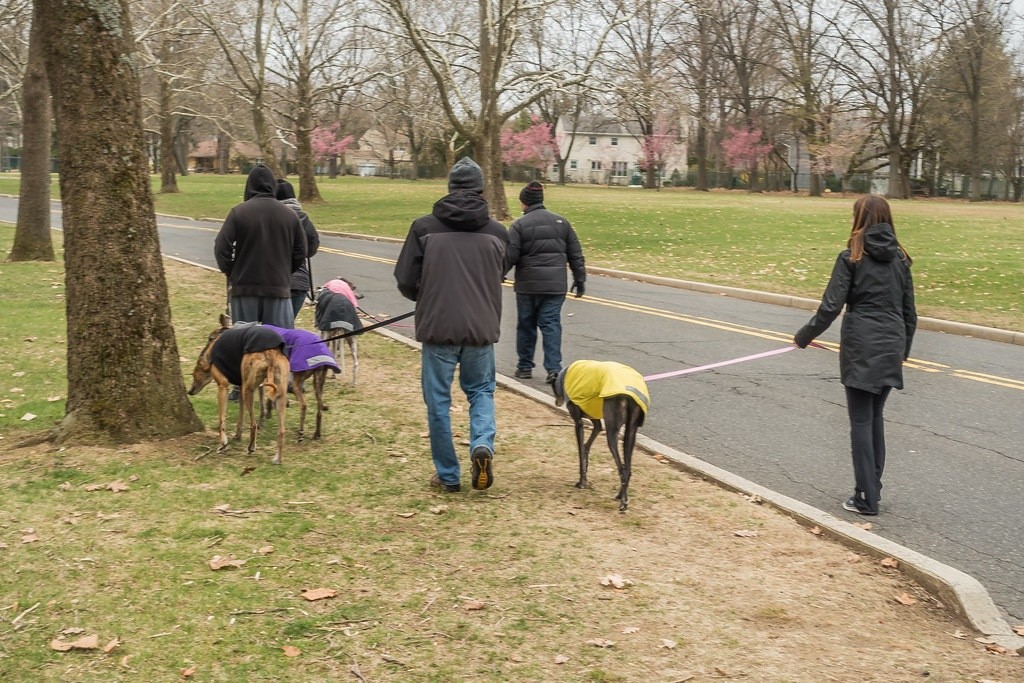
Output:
[470,447,493,490]
[227,385,239,400]
[287,382,305,395]
[842,497,877,514]
[515,368,532,378]
[430,473,460,493]
[547,372,557,383]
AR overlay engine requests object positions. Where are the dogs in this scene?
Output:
[315,276,364,386]
[187,314,328,463]
[545,359,651,510]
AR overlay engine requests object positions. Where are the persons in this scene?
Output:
[393,157,510,492]
[502,180,586,383]
[214,165,320,401]
[792,195,918,514]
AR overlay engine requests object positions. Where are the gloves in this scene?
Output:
[571,281,585,298]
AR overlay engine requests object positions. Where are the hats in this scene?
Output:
[520,181,544,205]
[448,156,484,195]
